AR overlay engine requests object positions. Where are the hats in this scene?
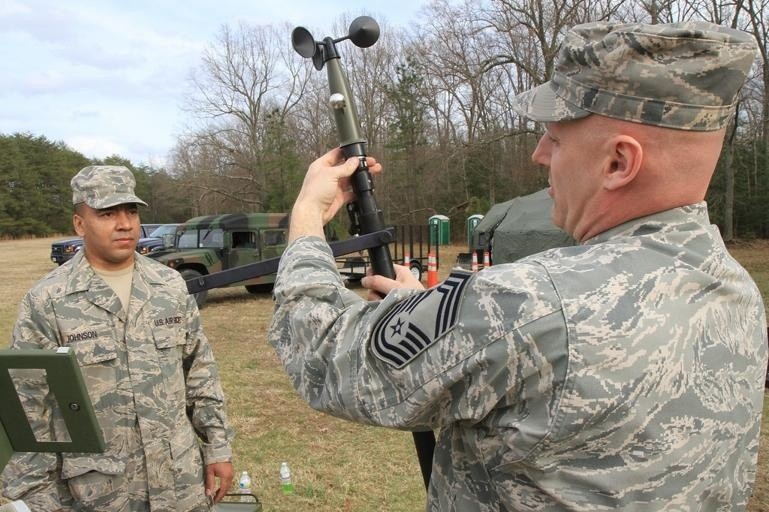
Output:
[514,22,758,132]
[70,165,149,210]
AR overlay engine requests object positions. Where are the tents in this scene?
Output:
[472,188,577,266]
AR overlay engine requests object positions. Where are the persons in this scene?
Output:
[0,167,235,512]
[269,20,768,512]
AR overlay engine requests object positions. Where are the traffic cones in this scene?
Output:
[404,250,411,269]
[427,250,437,288]
[483,250,490,267]
[472,250,478,273]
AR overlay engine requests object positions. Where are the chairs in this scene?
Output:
[244,242,256,249]
[263,231,286,245]
[236,244,244,248]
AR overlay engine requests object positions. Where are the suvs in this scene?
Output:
[50,211,291,310]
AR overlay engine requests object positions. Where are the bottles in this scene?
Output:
[239,471,251,502]
[280,462,293,496]
